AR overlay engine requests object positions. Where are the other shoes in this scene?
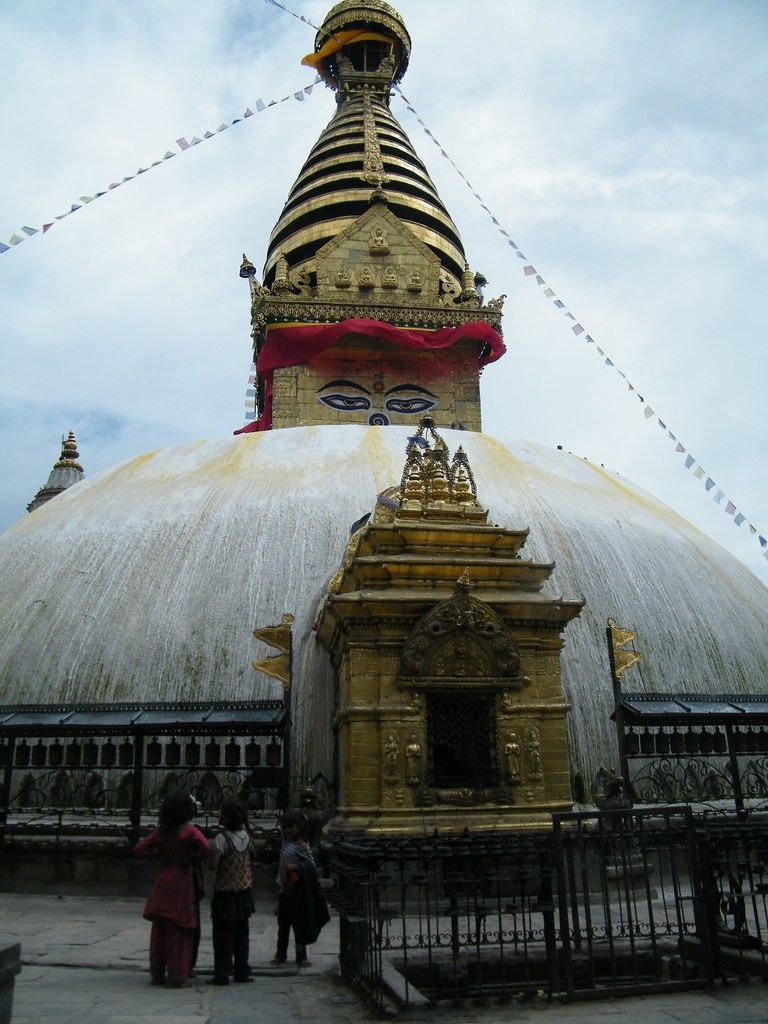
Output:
[303,961,312,968]
[207,978,229,985]
[270,958,283,966]
[234,976,254,983]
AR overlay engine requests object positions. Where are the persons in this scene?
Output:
[133,790,212,989]
[502,692,512,708]
[411,693,422,708]
[384,734,420,779]
[370,229,388,248]
[405,644,521,678]
[204,796,257,985]
[506,732,541,777]
[271,808,316,967]
[336,267,422,290]
